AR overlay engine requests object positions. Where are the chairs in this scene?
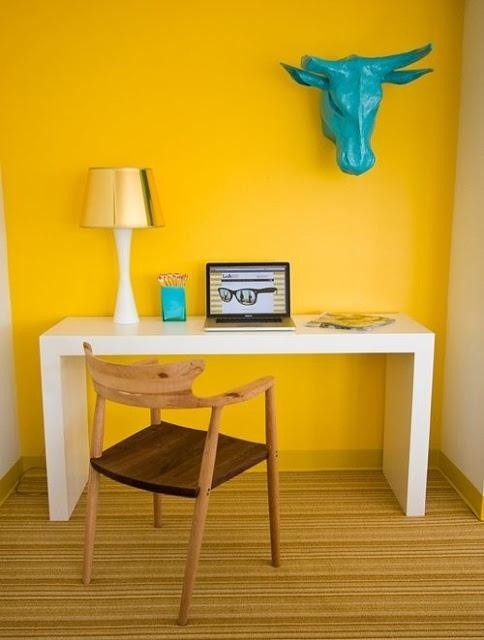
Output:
[81,342,281,626]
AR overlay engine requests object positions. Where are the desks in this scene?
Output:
[39,313,436,521]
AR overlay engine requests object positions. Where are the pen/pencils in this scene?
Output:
[158,272,188,288]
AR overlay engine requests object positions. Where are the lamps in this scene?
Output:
[79,167,166,324]
